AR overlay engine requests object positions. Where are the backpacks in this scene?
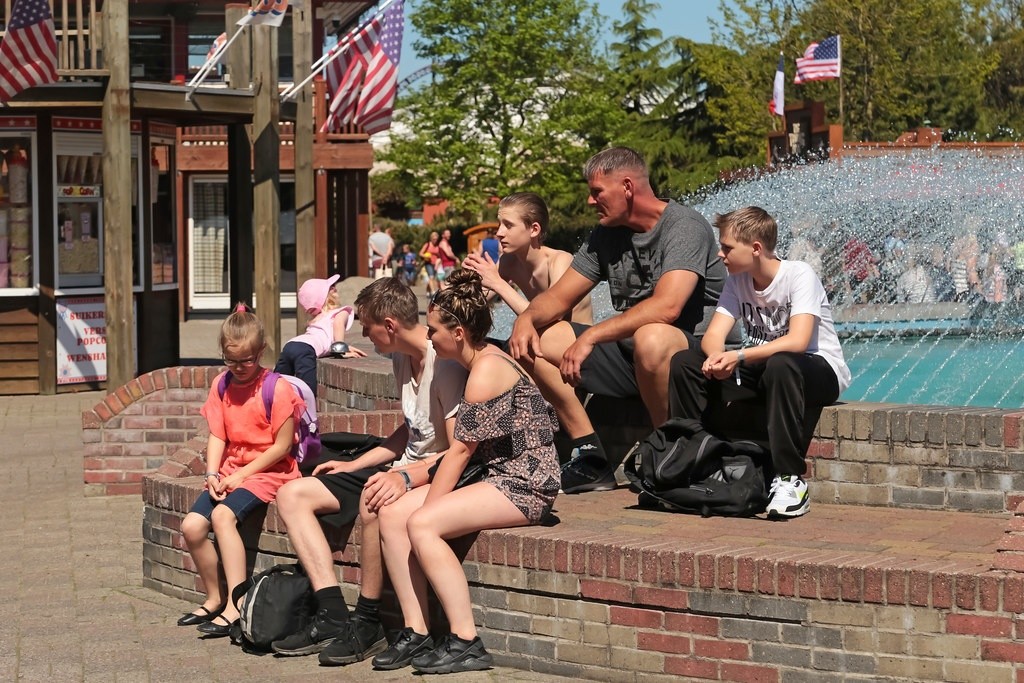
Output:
[218,370,321,463]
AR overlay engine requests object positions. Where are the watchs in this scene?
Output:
[204,472,220,481]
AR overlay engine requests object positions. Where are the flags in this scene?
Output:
[0,0,59,102]
[773,52,784,116]
[793,36,840,84]
[208,0,288,63]
[320,0,405,135]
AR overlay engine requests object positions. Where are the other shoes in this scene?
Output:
[427,290,435,298]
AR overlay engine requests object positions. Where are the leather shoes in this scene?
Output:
[196,614,241,635]
[176,603,227,625]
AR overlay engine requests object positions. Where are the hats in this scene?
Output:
[297,274,341,316]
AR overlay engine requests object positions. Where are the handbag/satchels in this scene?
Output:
[624,417,770,519]
[231,564,317,654]
[299,432,386,480]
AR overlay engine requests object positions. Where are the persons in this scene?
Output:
[274,275,367,399]
[509,146,728,493]
[460,193,593,404]
[372,268,561,674]
[777,202,1024,304]
[178,303,308,634]
[667,206,852,518]
[271,277,467,664]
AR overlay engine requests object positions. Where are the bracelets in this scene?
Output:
[737,349,744,366]
[398,470,411,491]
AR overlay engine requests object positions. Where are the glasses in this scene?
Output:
[222,354,258,367]
[429,291,461,328]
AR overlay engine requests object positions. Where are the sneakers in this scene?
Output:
[272,612,353,655]
[371,630,446,670]
[317,617,390,663]
[764,472,811,517]
[557,455,618,493]
[411,634,494,674]
